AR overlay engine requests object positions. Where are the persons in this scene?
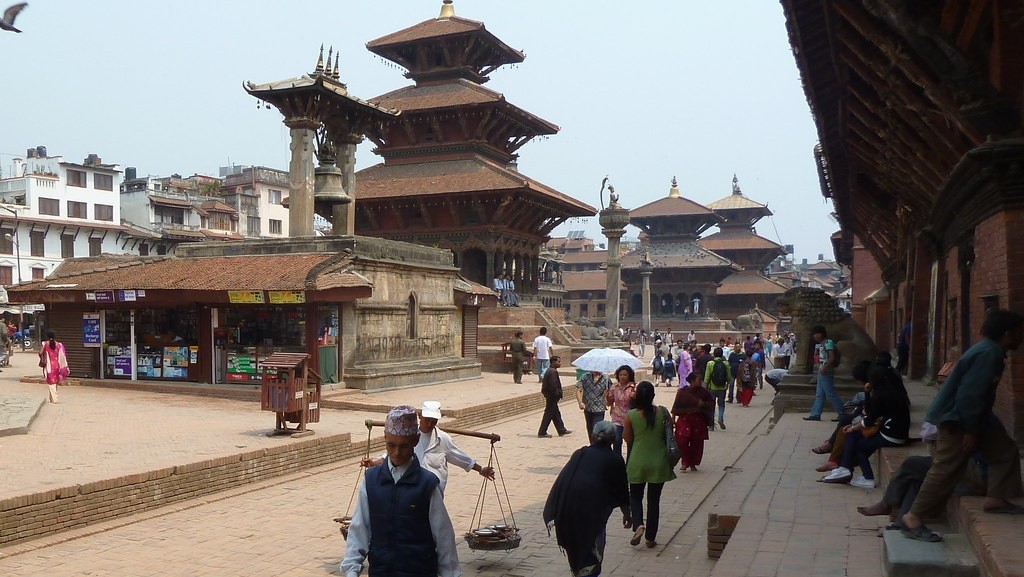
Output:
[339,275,1023,577]
[39,330,70,404]
[0,315,17,372]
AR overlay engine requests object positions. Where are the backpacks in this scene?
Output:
[824,338,841,368]
[712,359,728,386]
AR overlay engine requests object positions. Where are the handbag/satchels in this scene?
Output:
[661,406,680,462]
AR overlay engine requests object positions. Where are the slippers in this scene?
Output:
[900,525,943,542]
[983,500,1024,514]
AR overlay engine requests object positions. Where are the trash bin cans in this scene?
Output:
[501,342,513,373]
[280,368,322,423]
[260,352,308,413]
[522,350,534,374]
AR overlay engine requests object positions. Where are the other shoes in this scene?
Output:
[709,425,714,431]
[802,415,820,421]
[680,465,690,470]
[817,475,827,482]
[691,466,697,471]
[645,540,657,548]
[559,430,572,436]
[718,420,726,430]
[737,401,741,404]
[726,400,733,402]
[831,417,840,421]
[811,443,833,454]
[745,405,748,408]
[538,434,553,438]
[816,463,838,472]
[629,525,645,546]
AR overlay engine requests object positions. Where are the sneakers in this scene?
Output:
[850,476,875,488]
[825,467,851,481]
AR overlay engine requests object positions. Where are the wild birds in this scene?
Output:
[0,3,28,33]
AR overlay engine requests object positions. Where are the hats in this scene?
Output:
[422,401,442,419]
[938,362,954,376]
[385,405,418,437]
